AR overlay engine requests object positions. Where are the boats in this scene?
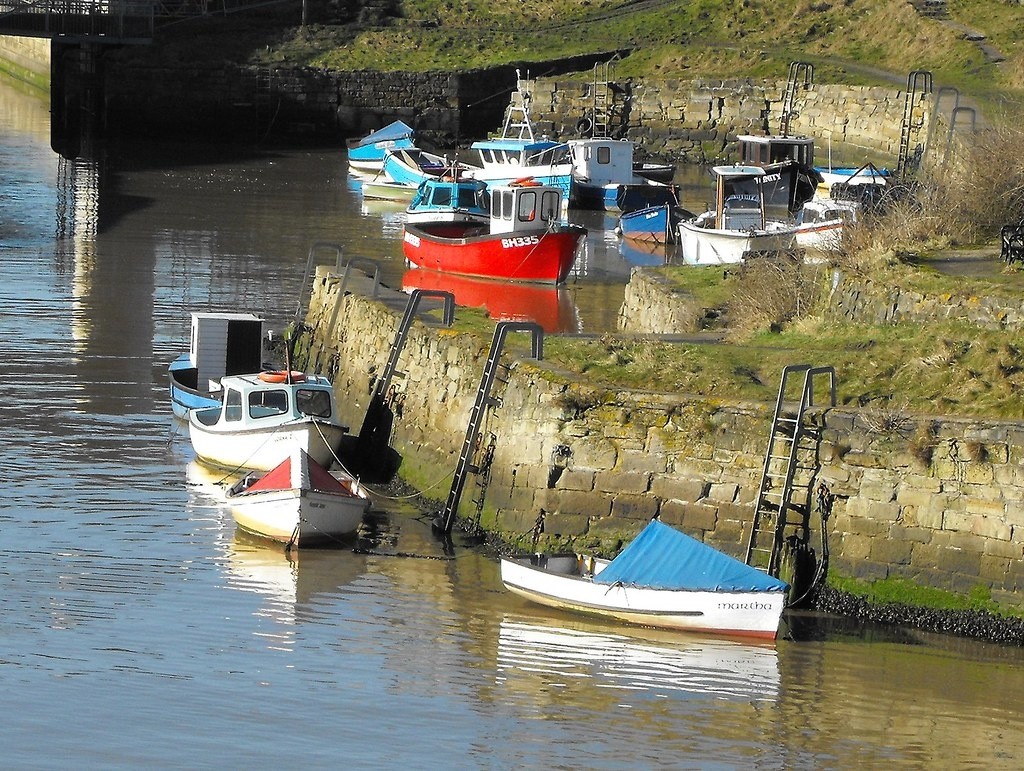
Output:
[383,146,483,188]
[401,157,590,284]
[497,515,793,641]
[344,119,417,172]
[468,134,899,268]
[166,309,351,474]
[225,448,372,548]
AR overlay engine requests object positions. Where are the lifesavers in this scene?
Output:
[260,368,305,383]
[575,117,593,134]
[508,175,543,186]
[443,176,467,183]
